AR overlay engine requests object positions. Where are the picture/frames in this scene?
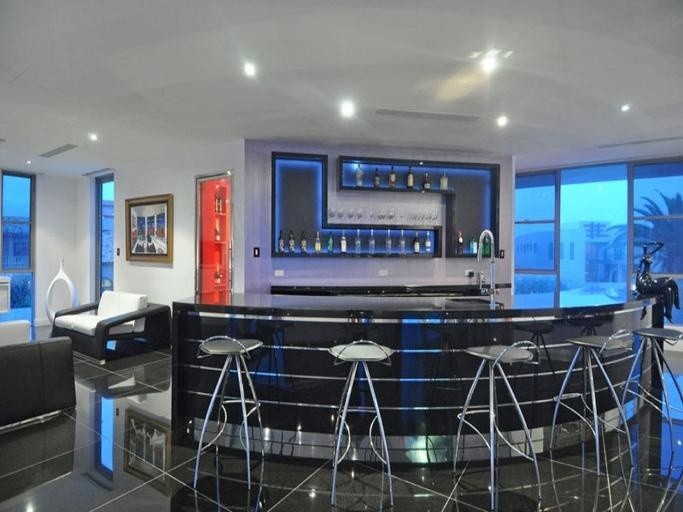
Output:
[120,408,172,496]
[124,193,173,265]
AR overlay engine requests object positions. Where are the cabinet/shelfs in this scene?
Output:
[263,149,510,295]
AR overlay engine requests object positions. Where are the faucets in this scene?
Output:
[477,229,496,308]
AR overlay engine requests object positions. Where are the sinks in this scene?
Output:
[434,297,504,310]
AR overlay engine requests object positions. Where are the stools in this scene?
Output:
[191,336,266,490]
[329,340,394,506]
[618,328,682,454]
[452,342,542,508]
[549,336,635,477]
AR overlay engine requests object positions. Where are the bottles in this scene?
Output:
[280,228,492,256]
[356,164,448,191]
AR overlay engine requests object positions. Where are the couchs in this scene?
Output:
[1,292,170,431]
